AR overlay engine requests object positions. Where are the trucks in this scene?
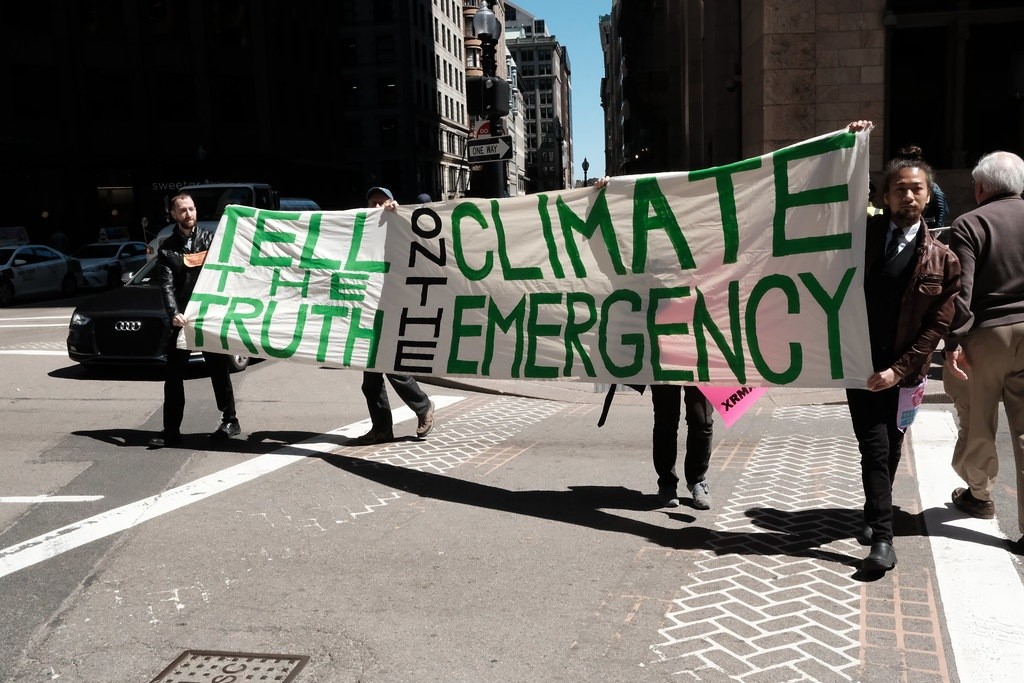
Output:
[143,182,325,263]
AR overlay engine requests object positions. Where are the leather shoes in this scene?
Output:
[1005,536,1024,555]
[861,542,897,569]
[859,505,873,545]
[952,487,994,519]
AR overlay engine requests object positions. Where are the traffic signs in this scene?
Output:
[466,134,516,164]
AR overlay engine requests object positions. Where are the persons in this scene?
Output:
[149,192,241,446]
[942,150,1024,555]
[868,184,884,217]
[899,145,947,239]
[595,176,713,510]
[358,187,431,444]
[51,226,68,240]
[845,120,962,571]
[415,193,433,203]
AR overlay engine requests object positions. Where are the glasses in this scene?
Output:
[972,181,977,187]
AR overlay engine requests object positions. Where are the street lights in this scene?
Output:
[581,157,590,188]
[472,0,504,199]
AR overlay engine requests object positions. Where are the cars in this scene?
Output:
[0,227,30,247]
[66,251,257,371]
[72,241,149,289]
[0,244,73,309]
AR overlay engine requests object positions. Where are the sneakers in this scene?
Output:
[358,426,394,444]
[659,487,679,507]
[148,428,183,447]
[417,401,435,436]
[210,422,241,438]
[686,481,711,509]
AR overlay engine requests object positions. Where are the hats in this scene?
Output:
[366,187,394,201]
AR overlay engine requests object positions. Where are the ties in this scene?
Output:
[885,228,903,262]
